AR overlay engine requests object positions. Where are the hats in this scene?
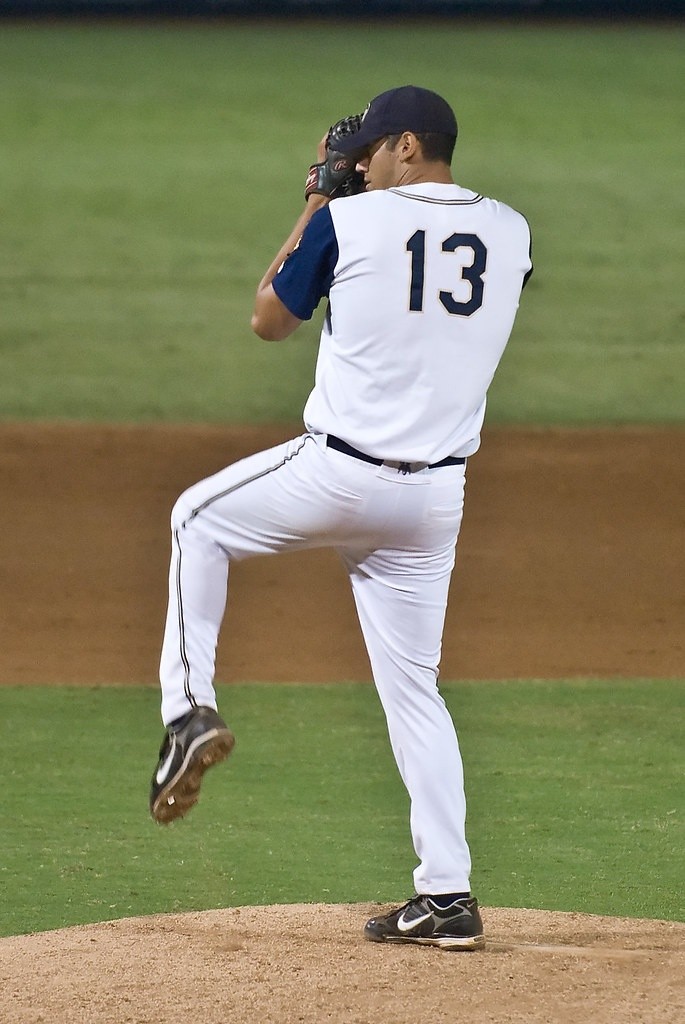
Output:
[329,84,459,151]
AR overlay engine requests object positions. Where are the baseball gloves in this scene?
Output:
[325,113,367,196]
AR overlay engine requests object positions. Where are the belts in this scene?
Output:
[326,435,465,469]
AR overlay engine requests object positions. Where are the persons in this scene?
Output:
[150,86,534,951]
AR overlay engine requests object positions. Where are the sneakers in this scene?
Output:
[149,705,234,823]
[364,891,486,950]
